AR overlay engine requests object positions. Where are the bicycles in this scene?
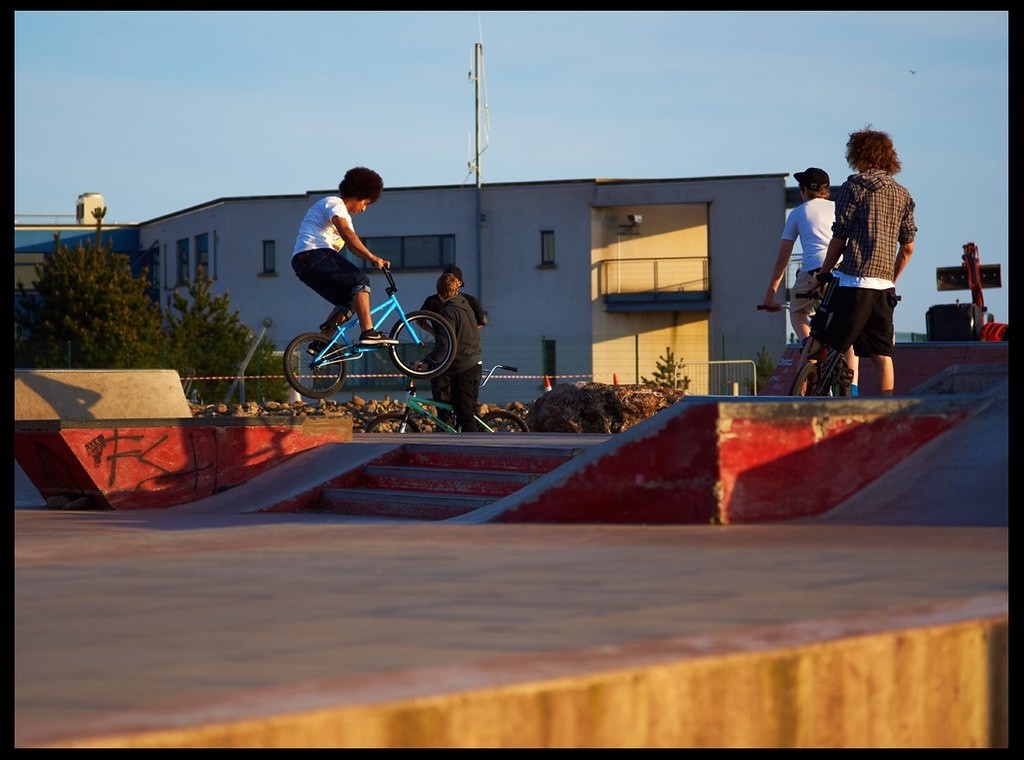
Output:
[282,261,458,399]
[366,363,531,433]
[756,291,855,399]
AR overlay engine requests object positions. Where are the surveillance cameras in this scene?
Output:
[626,214,643,223]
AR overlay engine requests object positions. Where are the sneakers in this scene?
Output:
[306,341,339,364]
[359,330,400,345]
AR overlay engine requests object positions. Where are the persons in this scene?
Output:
[289,167,400,357]
[765,131,859,397]
[800,131,917,397]
[413,267,488,432]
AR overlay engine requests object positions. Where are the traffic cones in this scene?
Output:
[543,375,552,397]
[612,372,619,386]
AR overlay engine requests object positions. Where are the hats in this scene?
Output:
[794,168,830,191]
[444,265,465,288]
